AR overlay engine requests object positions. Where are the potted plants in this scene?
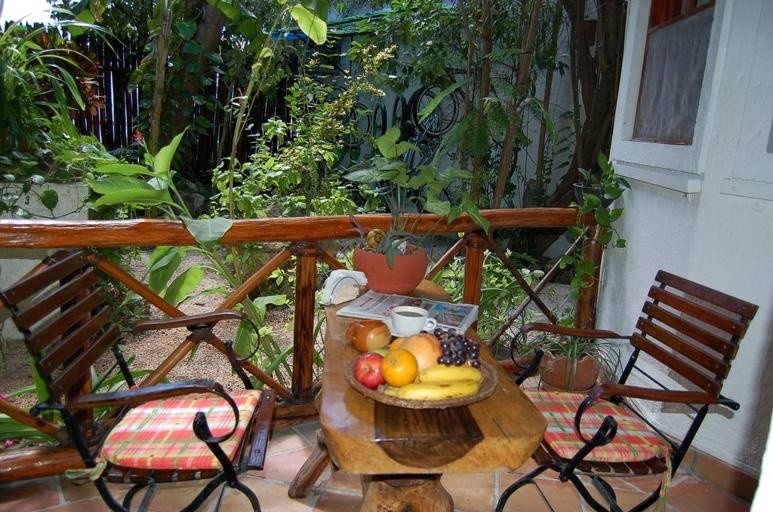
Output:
[343,127,490,295]
[518,332,624,392]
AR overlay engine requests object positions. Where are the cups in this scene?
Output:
[390,306,437,335]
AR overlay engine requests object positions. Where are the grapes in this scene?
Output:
[420,327,482,367]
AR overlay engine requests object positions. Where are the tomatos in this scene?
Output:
[354,353,387,390]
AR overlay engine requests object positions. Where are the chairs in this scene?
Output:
[0,249,277,512]
[494,270,759,512]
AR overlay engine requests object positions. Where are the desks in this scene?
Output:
[288,278,548,512]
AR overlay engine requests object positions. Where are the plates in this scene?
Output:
[346,318,497,410]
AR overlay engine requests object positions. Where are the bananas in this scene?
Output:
[417,365,484,382]
[397,379,482,400]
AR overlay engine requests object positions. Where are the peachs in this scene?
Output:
[401,333,443,368]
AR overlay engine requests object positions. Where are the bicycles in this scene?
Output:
[320,75,459,215]
